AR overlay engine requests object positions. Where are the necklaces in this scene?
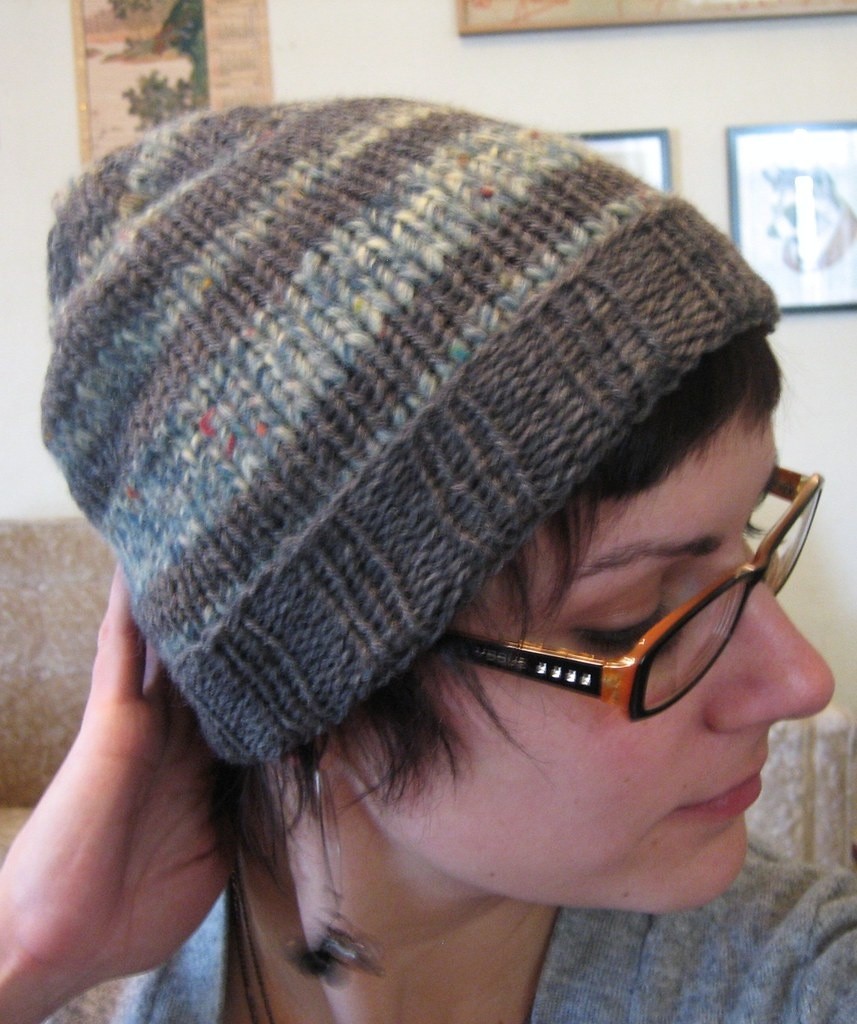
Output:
[228,872,275,1024]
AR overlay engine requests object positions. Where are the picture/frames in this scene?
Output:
[560,129,673,201]
[724,121,857,315]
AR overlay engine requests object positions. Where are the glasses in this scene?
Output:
[435,467,823,729]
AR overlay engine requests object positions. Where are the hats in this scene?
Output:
[41,97,777,767]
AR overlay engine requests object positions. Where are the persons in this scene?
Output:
[1,97,857,1024]
